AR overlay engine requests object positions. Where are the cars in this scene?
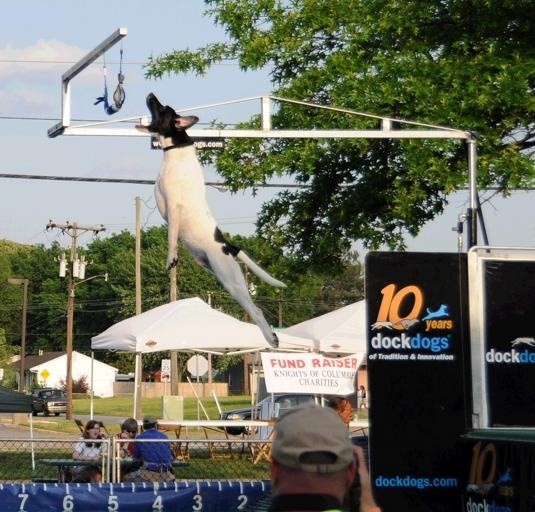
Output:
[31,388,67,416]
[115,370,155,382]
[217,393,330,436]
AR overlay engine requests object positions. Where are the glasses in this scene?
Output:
[127,429,136,434]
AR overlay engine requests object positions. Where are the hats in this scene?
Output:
[142,415,158,425]
[271,405,354,474]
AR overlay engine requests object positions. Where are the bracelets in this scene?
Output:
[105,434,109,439]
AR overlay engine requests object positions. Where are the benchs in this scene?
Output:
[31,476,76,483]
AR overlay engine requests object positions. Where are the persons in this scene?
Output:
[72,420,111,483]
[113,418,142,480]
[123,415,171,483]
[266,402,382,511]
[213,366,227,382]
[329,396,353,425]
[356,385,369,412]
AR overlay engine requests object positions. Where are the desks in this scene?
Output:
[122,418,269,462]
[38,458,192,483]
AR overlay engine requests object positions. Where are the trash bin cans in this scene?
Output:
[162,396,184,421]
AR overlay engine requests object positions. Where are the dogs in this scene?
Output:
[134,90,289,350]
